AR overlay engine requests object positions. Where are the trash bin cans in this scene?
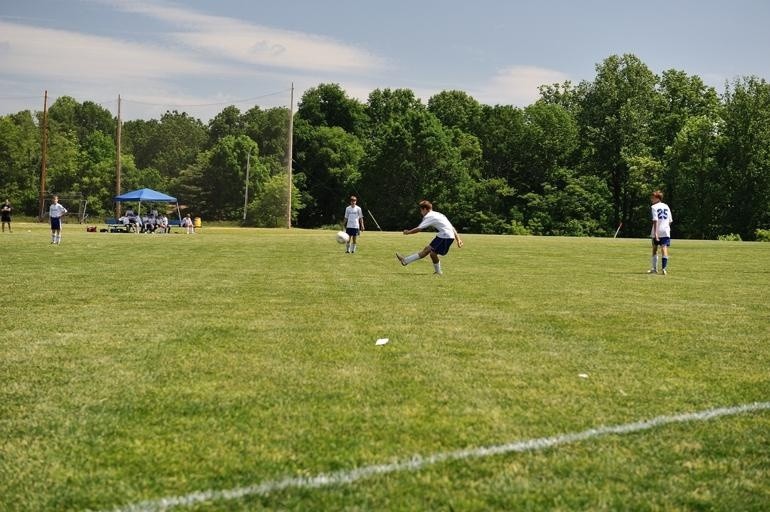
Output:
[194,217,202,228]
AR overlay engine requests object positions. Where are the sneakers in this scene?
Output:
[647,267,667,274]
[432,272,443,275]
[345,251,355,253]
[396,252,408,266]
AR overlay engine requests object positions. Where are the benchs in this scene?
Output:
[108,223,171,233]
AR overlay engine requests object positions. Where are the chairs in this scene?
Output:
[179,222,194,234]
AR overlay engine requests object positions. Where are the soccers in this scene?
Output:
[336,231,350,245]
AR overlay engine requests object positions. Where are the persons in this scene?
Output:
[393,200,464,276]
[341,195,365,254]
[117,205,195,234]
[48,195,68,244]
[646,188,674,277]
[1,198,13,233]
